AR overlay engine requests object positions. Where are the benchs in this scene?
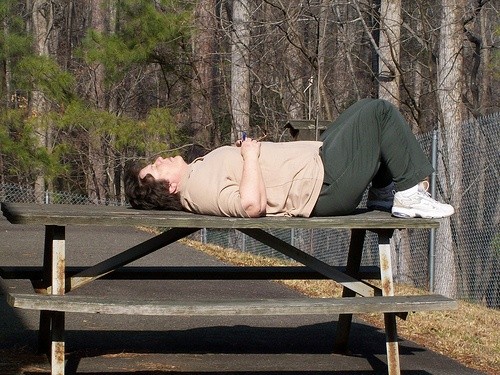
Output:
[5,290,458,375]
[0,265,382,346]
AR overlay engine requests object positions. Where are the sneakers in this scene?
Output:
[365,182,399,210]
[392,184,456,219]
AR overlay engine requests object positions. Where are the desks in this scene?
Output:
[0,195,441,375]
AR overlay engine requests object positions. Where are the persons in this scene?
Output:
[131,100,455,220]
